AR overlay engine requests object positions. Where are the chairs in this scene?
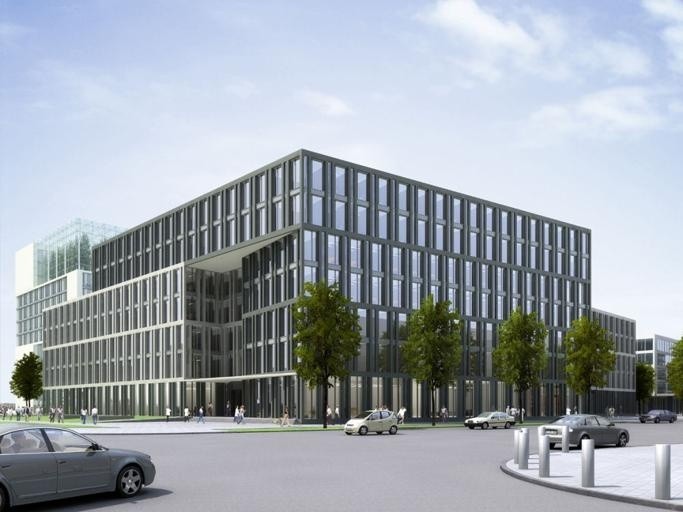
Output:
[1,437,40,455]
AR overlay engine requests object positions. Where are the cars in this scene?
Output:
[540,414,629,449]
[639,409,677,423]
[464,411,515,430]
[344,409,398,435]
[0,420,155,512]
